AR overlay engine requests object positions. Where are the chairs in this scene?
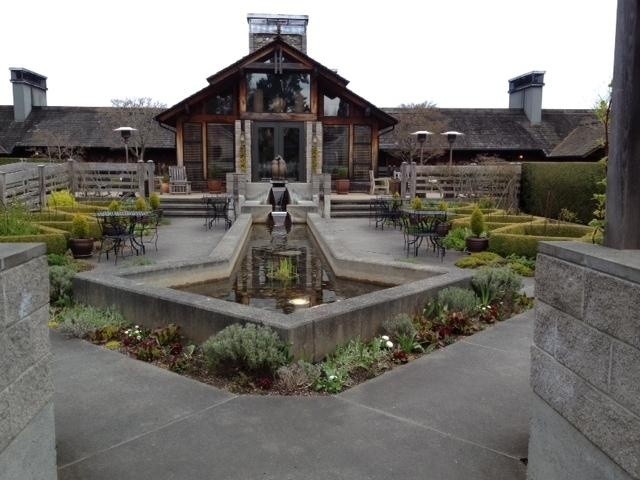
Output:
[202,193,233,231]
[169,165,192,195]
[96,209,163,265]
[369,195,405,231]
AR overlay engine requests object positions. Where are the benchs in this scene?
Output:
[369,170,389,195]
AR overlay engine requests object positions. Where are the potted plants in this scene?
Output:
[336,165,350,194]
[409,196,429,226]
[207,159,223,194]
[436,200,450,237]
[104,191,163,236]
[465,206,489,255]
[69,213,94,259]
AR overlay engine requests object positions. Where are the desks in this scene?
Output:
[402,209,457,256]
